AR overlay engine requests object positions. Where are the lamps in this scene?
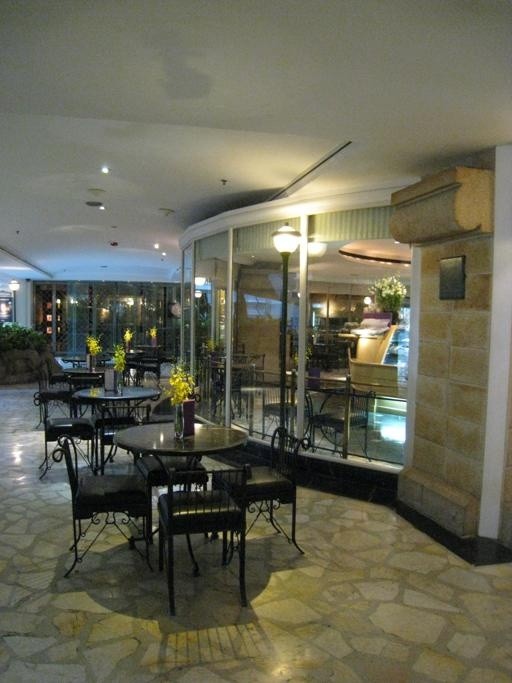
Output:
[7,280,20,290]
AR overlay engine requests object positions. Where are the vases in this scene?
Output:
[384,311,399,325]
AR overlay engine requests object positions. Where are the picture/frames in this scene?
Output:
[0,289,16,326]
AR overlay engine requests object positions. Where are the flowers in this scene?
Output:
[124,328,135,346]
[366,268,407,312]
[149,325,157,337]
[86,333,105,368]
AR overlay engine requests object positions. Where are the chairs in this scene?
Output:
[157,463,247,617]
[141,393,202,425]
[95,404,151,475]
[33,392,96,479]
[24,341,181,391]
[53,434,156,577]
[30,370,77,430]
[212,427,305,563]
[181,340,376,461]
[135,420,209,546]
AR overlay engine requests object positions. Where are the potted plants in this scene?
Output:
[164,365,196,438]
